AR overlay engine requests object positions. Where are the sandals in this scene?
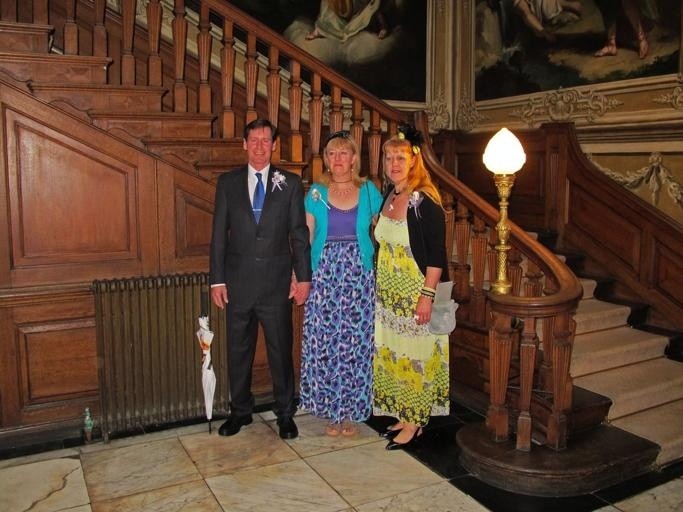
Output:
[379,26,389,40]
[306,32,324,40]
[637,31,649,59]
[594,37,616,57]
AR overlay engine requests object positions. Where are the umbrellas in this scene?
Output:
[195,272,219,438]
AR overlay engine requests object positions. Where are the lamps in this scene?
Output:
[482,126,526,296]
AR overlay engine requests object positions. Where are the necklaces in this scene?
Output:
[328,180,354,194]
[387,188,401,211]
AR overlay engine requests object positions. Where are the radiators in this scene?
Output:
[83,272,230,444]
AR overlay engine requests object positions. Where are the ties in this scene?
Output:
[253,173,266,224]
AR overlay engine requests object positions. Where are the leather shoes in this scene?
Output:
[219,414,253,436]
[276,419,298,439]
[327,421,340,436]
[386,427,423,450]
[380,426,403,437]
[339,421,355,436]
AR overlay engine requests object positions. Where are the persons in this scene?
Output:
[289,129,384,437]
[594,0,657,59]
[506,0,582,42]
[210,119,313,440]
[305,0,389,40]
[371,126,456,450]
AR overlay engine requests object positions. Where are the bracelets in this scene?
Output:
[421,287,435,299]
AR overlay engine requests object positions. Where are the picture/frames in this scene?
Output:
[185,0,454,134]
[453,0,683,142]
